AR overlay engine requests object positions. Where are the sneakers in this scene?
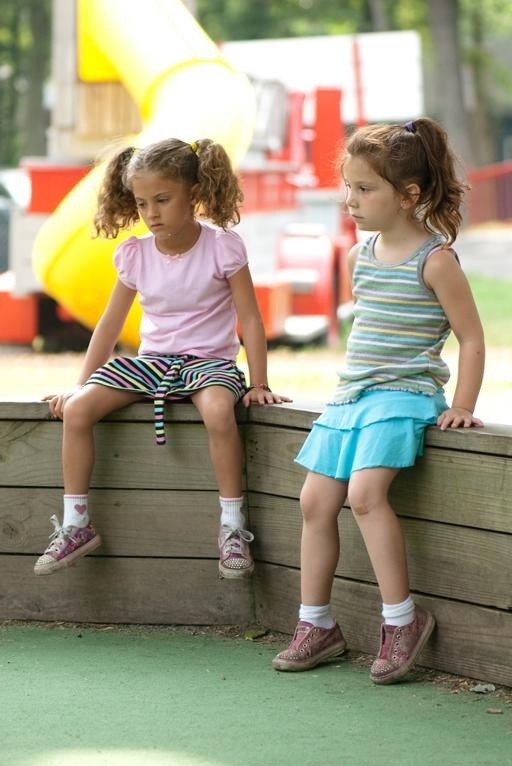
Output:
[270,616,348,672]
[32,518,102,577]
[368,601,438,684]
[216,520,258,581]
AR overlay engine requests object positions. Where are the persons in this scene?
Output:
[272,118,487,685]
[32,137,294,582]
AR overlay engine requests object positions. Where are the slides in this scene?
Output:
[30,0,256,350]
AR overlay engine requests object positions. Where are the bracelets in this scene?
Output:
[248,383,271,392]
